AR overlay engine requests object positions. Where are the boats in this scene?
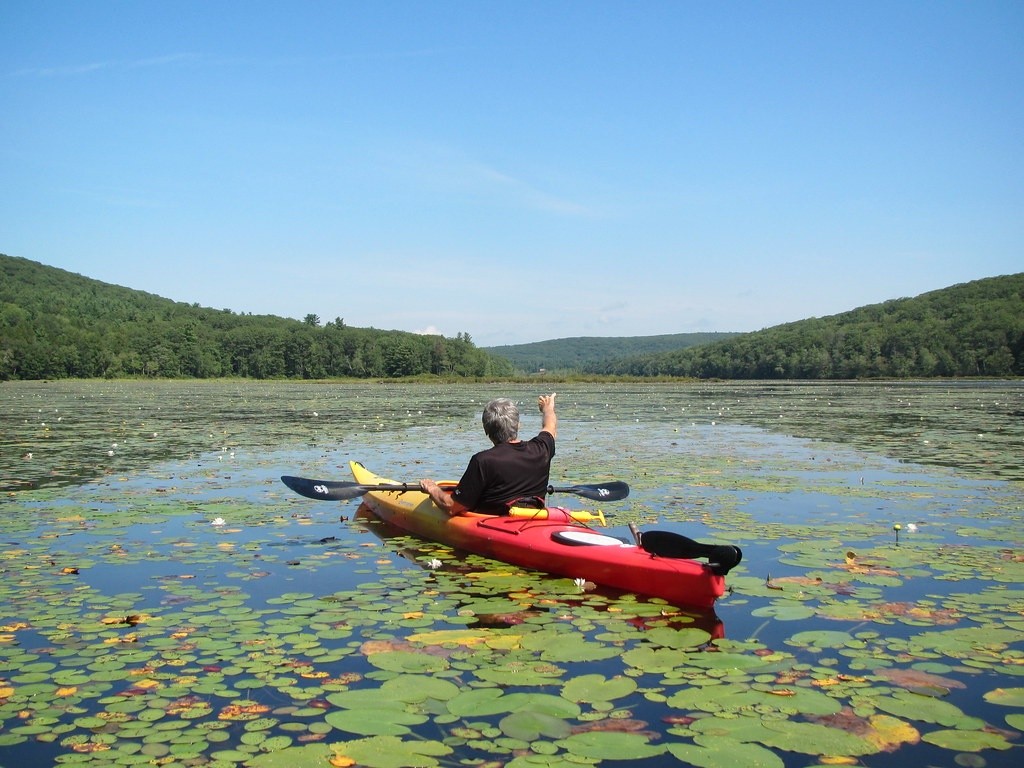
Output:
[350,458,744,614]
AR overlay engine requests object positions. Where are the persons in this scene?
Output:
[421,393,558,516]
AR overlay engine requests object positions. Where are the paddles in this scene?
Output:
[280,475,630,502]
